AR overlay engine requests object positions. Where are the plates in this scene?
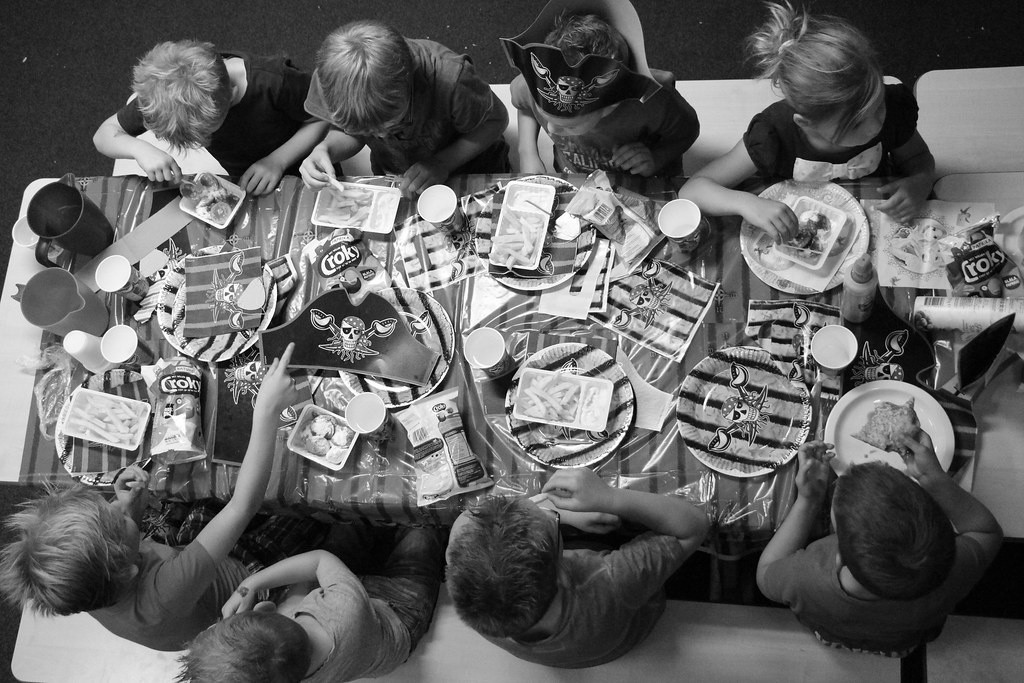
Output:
[676,345,812,477]
[506,344,639,468]
[475,175,599,291]
[335,287,455,410]
[821,380,955,490]
[740,178,870,296]
[157,245,277,363]
[813,324,859,371]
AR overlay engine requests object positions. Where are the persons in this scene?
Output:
[0,343,316,652]
[92,40,332,197]
[174,526,443,683]
[755,428,1004,658]
[676,0,938,245]
[444,467,710,670]
[499,1,701,176]
[299,20,511,195]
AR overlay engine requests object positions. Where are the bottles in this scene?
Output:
[913,298,1024,332]
[842,253,879,325]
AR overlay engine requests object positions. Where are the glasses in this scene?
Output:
[538,506,561,556]
[343,70,416,138]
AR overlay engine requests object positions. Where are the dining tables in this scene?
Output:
[0,172,1024,543]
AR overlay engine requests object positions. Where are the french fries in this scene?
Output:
[320,189,371,226]
[490,211,543,270]
[521,377,580,422]
[71,398,146,446]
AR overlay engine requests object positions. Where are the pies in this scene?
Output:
[853,398,919,453]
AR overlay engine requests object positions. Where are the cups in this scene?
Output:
[63,330,117,375]
[417,183,464,234]
[96,254,150,302]
[344,393,395,443]
[464,326,508,379]
[100,324,154,370]
[26,173,116,275]
[11,268,109,338]
[658,198,703,252]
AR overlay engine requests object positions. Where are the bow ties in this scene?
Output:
[792,142,883,185]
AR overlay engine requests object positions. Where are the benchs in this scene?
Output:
[11,576,1024,683]
[332,64,1024,214]
